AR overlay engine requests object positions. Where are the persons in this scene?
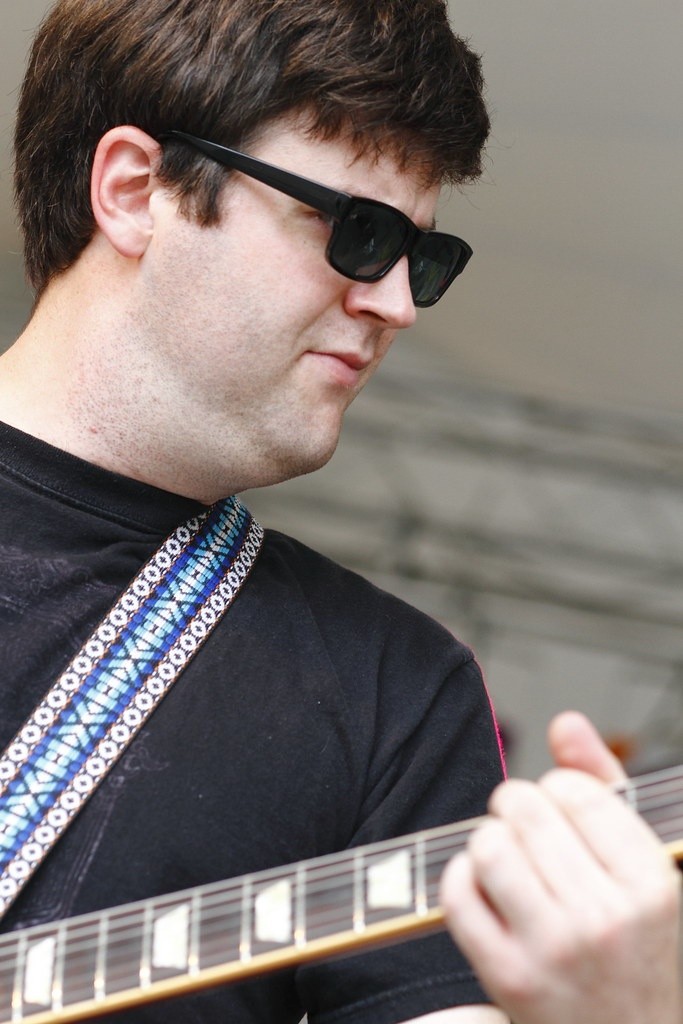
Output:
[0,0,683,1024]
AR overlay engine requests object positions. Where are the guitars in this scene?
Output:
[0,759,679,1024]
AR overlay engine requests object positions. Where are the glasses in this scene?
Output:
[167,130,473,308]
